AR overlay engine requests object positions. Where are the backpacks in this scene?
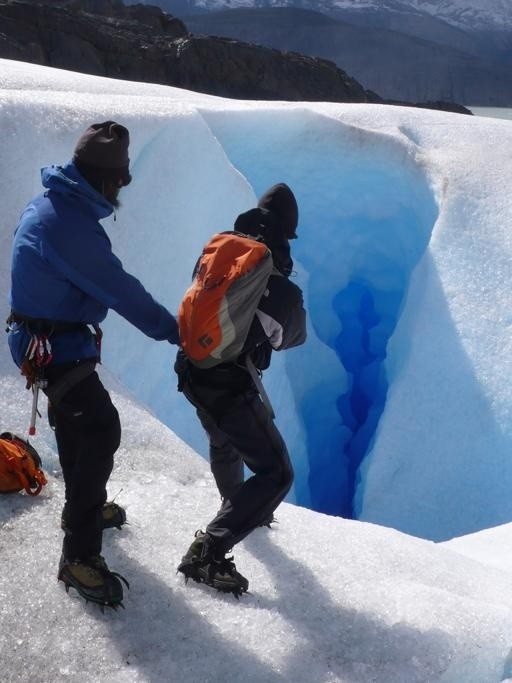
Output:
[0,431,49,495]
[177,230,274,370]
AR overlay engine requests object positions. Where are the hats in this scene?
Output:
[258,182,298,240]
[74,119,133,188]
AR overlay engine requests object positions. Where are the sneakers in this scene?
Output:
[218,498,276,529]
[60,502,128,535]
[57,550,124,604]
[175,533,249,594]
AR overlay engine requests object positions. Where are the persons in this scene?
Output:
[4,120,178,617]
[171,181,307,593]
